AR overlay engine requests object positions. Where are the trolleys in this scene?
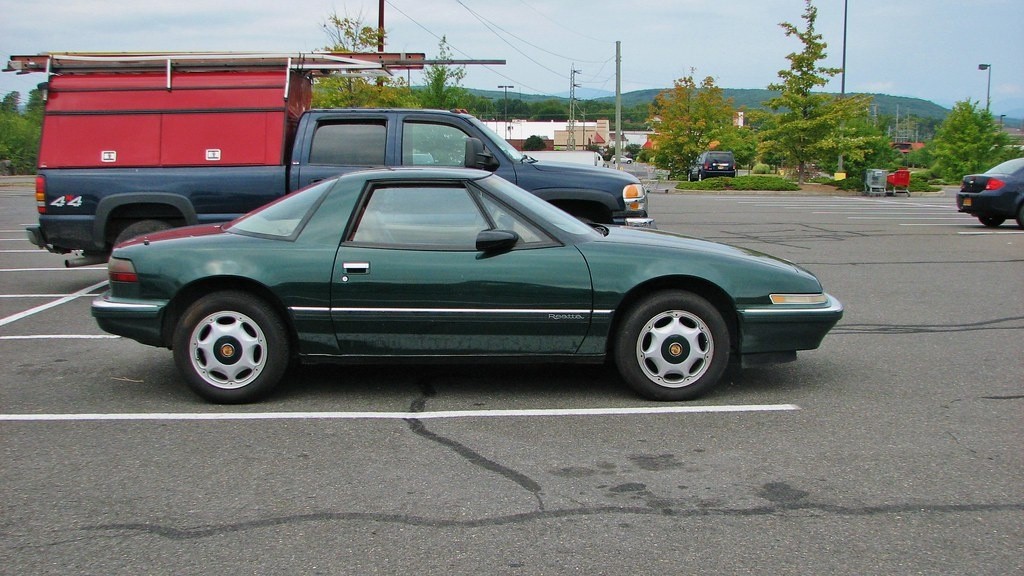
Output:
[887,170,911,197]
[863,169,888,197]
[643,163,670,193]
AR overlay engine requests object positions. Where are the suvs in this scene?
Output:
[687,150,736,180]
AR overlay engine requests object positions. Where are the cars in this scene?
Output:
[956,158,1024,228]
[610,155,633,164]
[91,169,844,405]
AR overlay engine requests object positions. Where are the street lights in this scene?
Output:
[978,62,991,114]
[498,85,514,141]
[480,96,494,126]
[999,114,1007,131]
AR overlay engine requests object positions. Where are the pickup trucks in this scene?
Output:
[25,107,654,268]
